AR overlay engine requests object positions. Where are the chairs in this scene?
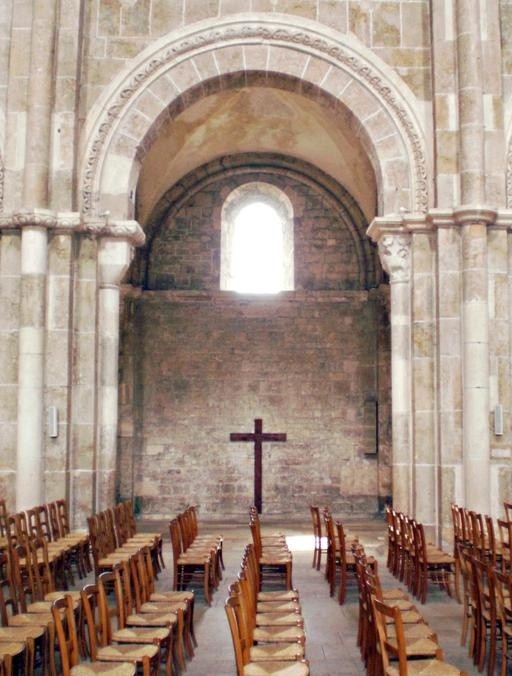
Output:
[0,489,224,675]
[225,499,510,675]
